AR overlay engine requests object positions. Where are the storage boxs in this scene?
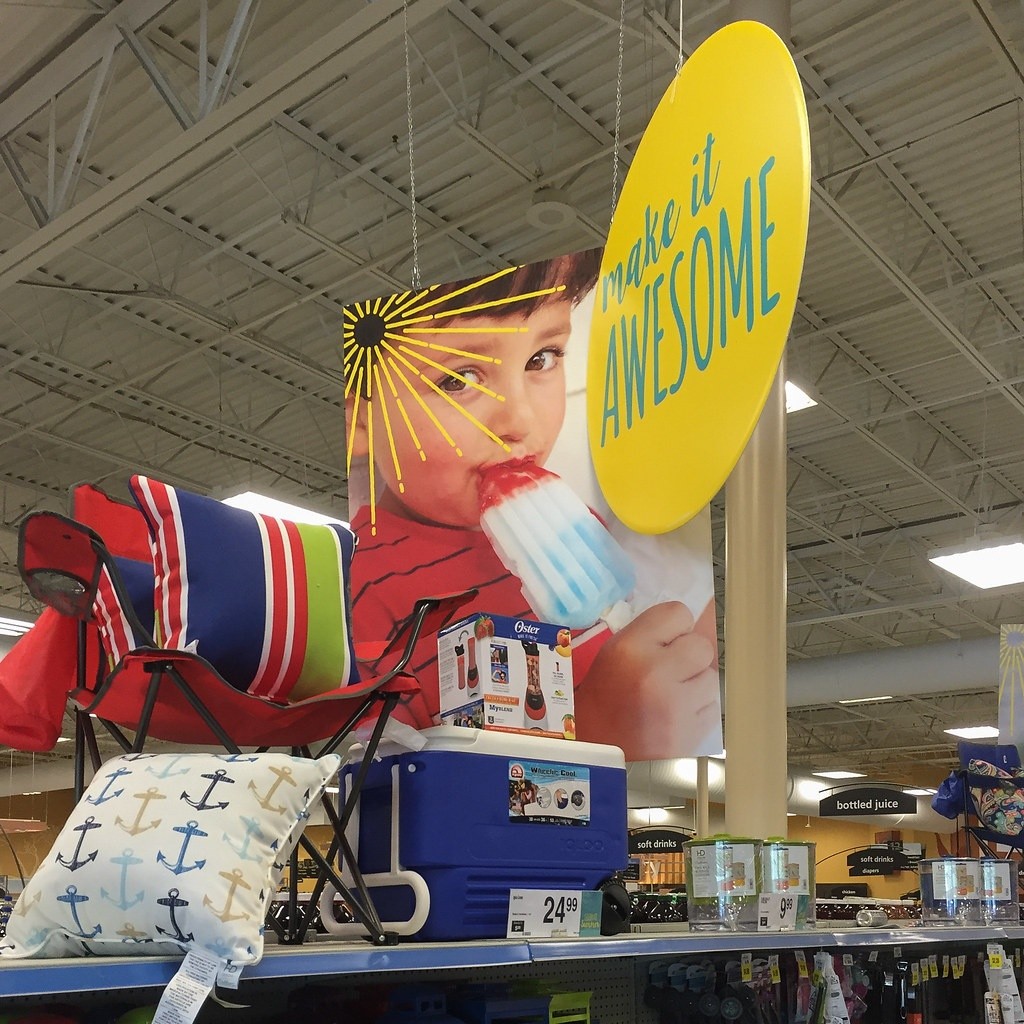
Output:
[318,614,629,939]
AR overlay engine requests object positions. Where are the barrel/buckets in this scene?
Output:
[916,855,1019,927]
[681,834,816,934]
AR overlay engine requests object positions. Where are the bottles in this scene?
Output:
[814,898,921,920]
[628,893,688,923]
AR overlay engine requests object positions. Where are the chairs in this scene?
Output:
[0,480,479,945]
[931,741,1024,891]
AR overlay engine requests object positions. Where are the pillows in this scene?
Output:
[967,758,1024,837]
[0,753,341,1024]
[73,475,361,703]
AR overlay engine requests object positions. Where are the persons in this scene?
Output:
[348,249,722,760]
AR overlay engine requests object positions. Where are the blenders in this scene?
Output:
[521,639,548,731]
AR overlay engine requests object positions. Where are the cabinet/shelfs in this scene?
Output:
[1,924,1024,1024]
